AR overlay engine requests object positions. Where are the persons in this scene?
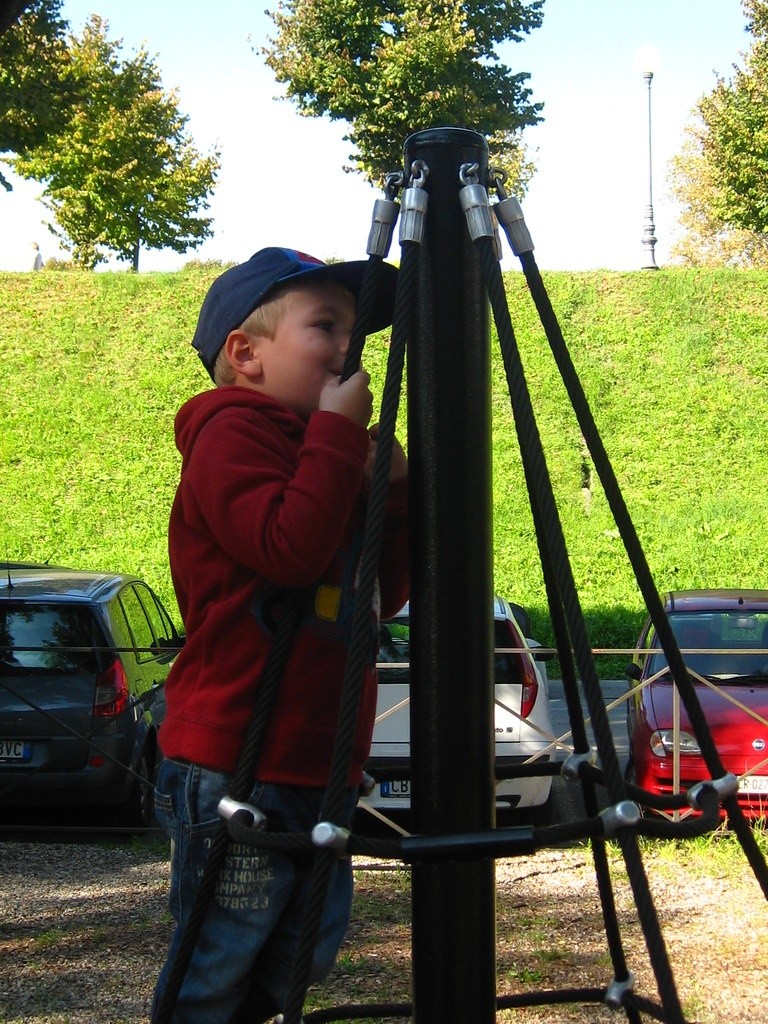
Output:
[143,247,410,1024]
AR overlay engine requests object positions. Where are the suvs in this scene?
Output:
[362,589,556,842]
[0,568,189,836]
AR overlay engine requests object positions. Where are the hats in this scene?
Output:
[191,247,400,382]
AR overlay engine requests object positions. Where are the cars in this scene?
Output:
[623,588,768,833]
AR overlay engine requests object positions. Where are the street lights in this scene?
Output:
[640,69,660,271]
